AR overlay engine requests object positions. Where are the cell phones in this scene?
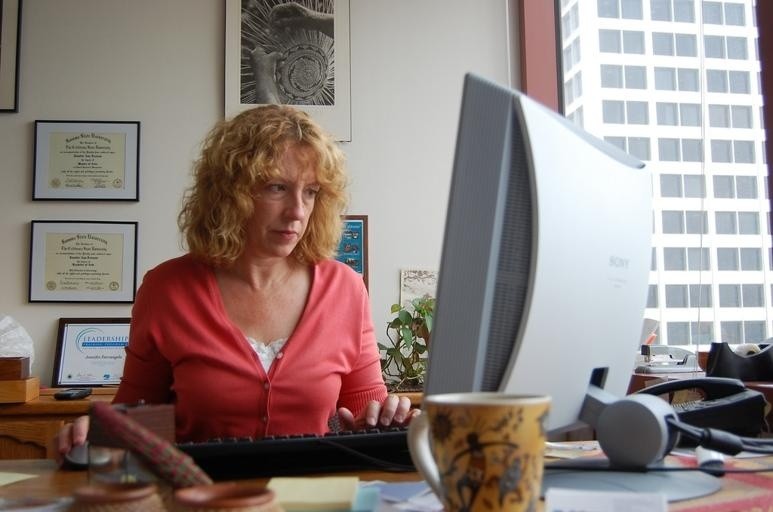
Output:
[55,388,93,400]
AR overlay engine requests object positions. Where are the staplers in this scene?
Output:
[634,344,699,373]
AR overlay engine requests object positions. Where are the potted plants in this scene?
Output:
[377,293,434,393]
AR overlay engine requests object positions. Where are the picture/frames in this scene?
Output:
[335,215,368,296]
[28,221,137,303]
[398,266,438,358]
[51,318,132,388]
[225,0,350,142]
[0,0,22,112]
[31,120,140,201]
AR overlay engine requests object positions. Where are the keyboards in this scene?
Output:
[175,425,418,483]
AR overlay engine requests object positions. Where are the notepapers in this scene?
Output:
[268,475,359,512]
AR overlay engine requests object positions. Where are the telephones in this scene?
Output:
[629,378,766,438]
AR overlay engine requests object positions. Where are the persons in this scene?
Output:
[57,104,422,454]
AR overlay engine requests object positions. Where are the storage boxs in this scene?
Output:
[0,358,30,380]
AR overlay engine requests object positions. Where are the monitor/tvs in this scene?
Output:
[422,71,682,469]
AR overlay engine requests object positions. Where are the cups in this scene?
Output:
[408,392,550,512]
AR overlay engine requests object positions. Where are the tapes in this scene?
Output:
[735,344,760,357]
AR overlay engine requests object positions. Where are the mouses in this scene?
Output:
[62,440,112,472]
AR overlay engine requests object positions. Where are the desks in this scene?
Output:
[624,367,773,433]
[1,441,771,512]
[0,390,119,459]
